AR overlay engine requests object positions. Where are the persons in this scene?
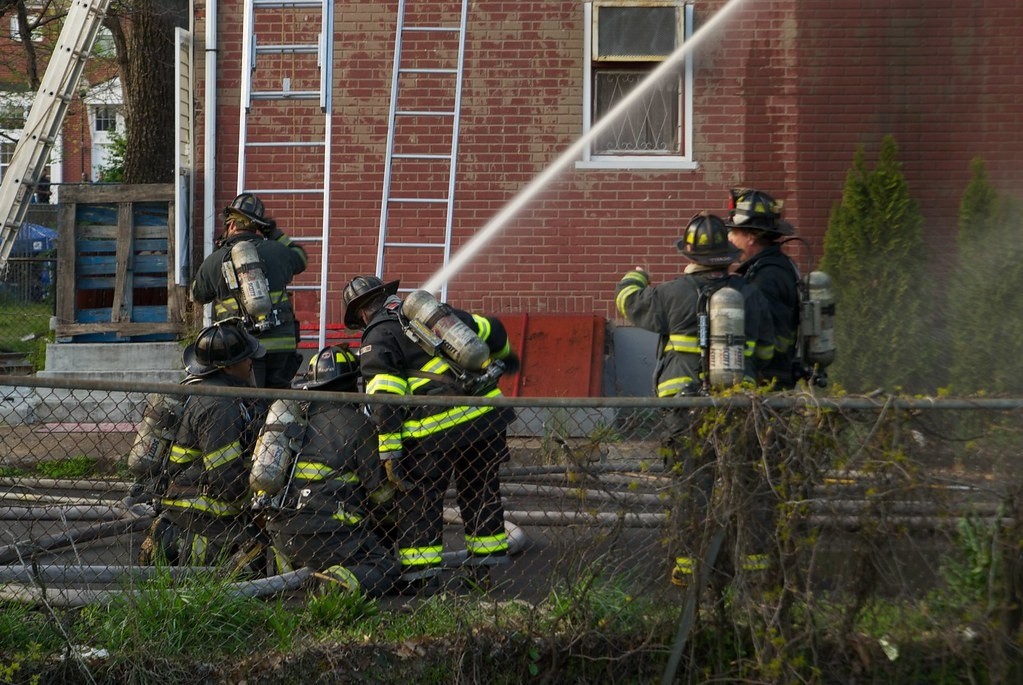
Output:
[616,190,810,634]
[139,193,520,621]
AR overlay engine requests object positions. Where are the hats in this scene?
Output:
[342,275,400,331]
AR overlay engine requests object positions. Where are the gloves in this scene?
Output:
[257,217,283,241]
[384,458,412,492]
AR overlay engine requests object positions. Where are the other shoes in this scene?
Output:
[417,577,440,596]
[466,567,491,589]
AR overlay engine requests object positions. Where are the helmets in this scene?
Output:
[290,345,360,389]
[677,212,739,266]
[222,192,270,226]
[725,190,795,235]
[181,324,259,376]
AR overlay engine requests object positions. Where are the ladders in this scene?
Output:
[236,0,334,352]
[375,0,467,304]
[0,0,109,275]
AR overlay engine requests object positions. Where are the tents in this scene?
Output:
[0,222,58,305]
